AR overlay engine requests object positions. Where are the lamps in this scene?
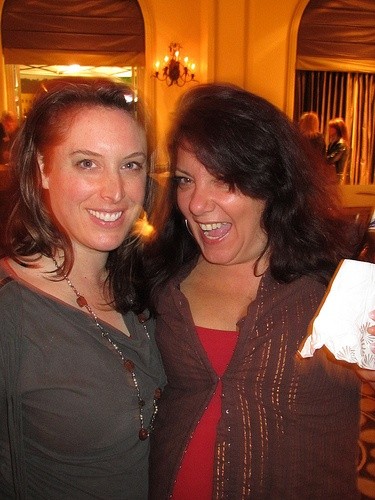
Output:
[152,42,200,87]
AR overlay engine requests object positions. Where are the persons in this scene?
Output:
[0,80,163,500]
[299,112,326,162]
[149,82,374,500]
[325,118,349,181]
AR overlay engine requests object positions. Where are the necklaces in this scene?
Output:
[49,252,162,441]
